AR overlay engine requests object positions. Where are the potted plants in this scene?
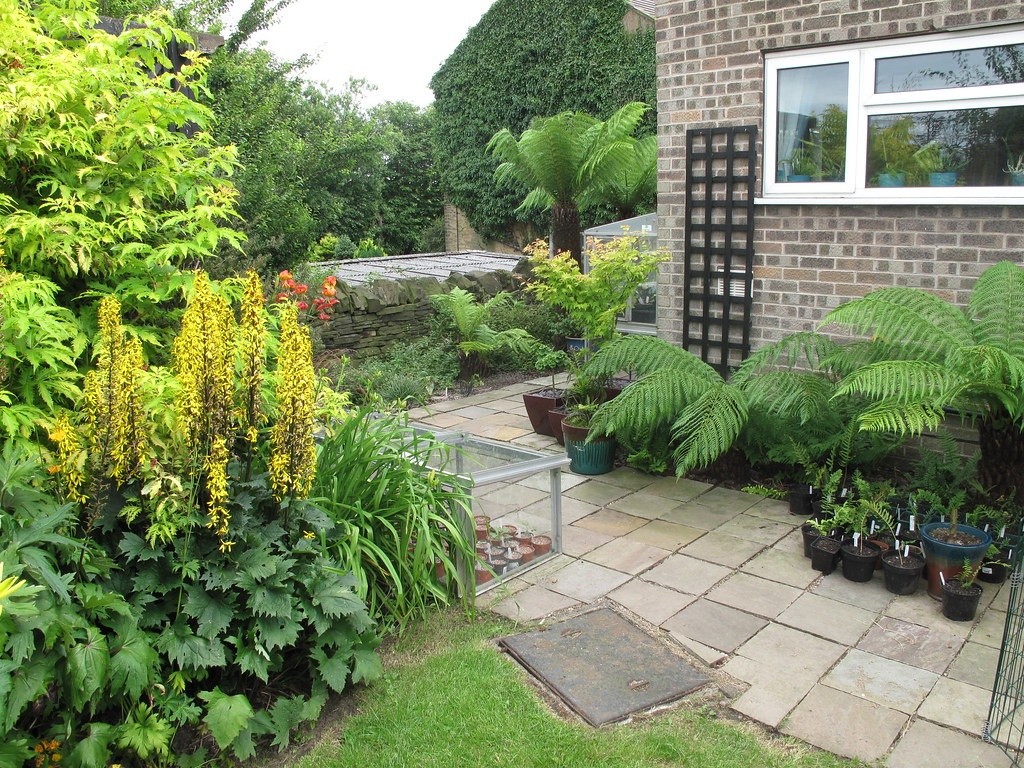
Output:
[1000,125,1024,187]
[785,427,1024,621]
[521,224,674,475]
[869,112,958,188]
[777,138,845,182]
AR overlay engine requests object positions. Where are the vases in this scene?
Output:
[404,509,552,585]
